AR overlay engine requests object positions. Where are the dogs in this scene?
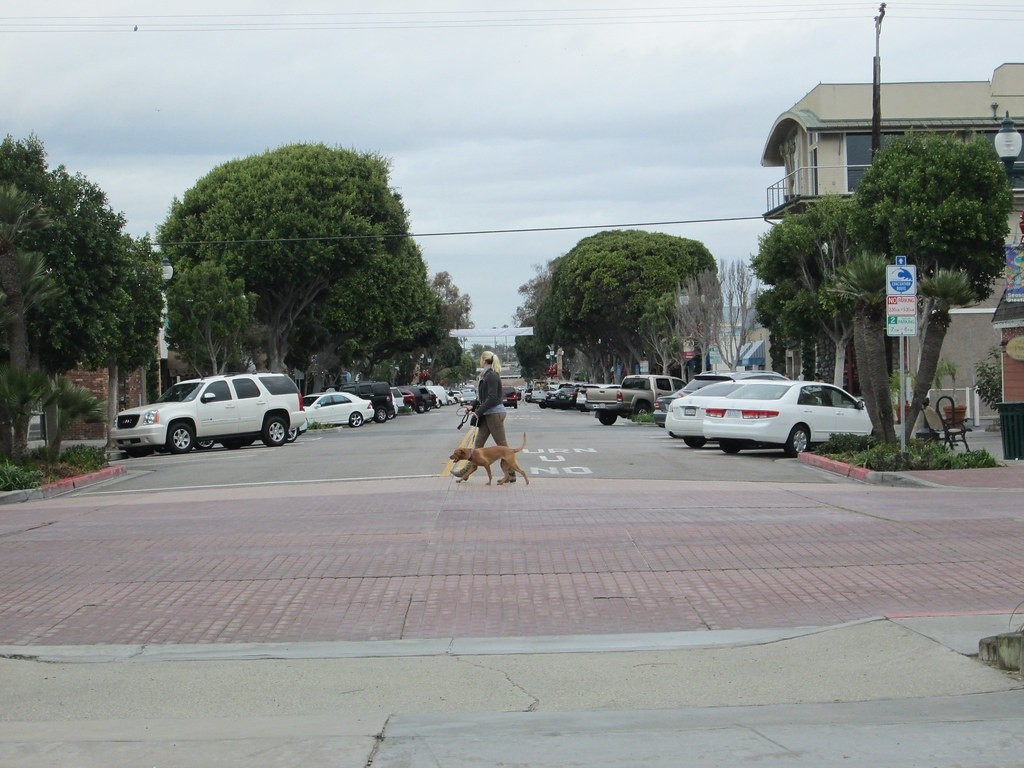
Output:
[450,432,530,486]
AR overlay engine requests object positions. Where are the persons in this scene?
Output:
[905,401,910,406]
[914,397,942,445]
[450,351,517,484]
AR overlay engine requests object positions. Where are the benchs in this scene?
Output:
[912,398,973,453]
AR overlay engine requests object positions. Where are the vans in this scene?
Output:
[500,385,518,409]
[319,382,396,424]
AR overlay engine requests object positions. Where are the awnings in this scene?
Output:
[735,340,766,366]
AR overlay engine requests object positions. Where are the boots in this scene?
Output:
[497,470,516,482]
[450,461,473,481]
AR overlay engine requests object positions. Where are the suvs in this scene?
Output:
[676,369,791,440]
[110,369,309,454]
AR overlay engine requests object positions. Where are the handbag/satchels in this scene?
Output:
[470,399,481,427]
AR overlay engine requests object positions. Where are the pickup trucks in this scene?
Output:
[584,374,688,426]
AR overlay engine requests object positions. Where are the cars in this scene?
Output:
[194,416,309,451]
[302,388,375,428]
[514,378,588,411]
[391,383,478,416]
[576,384,623,413]
[703,379,874,458]
[652,372,706,428]
[665,378,771,449]
